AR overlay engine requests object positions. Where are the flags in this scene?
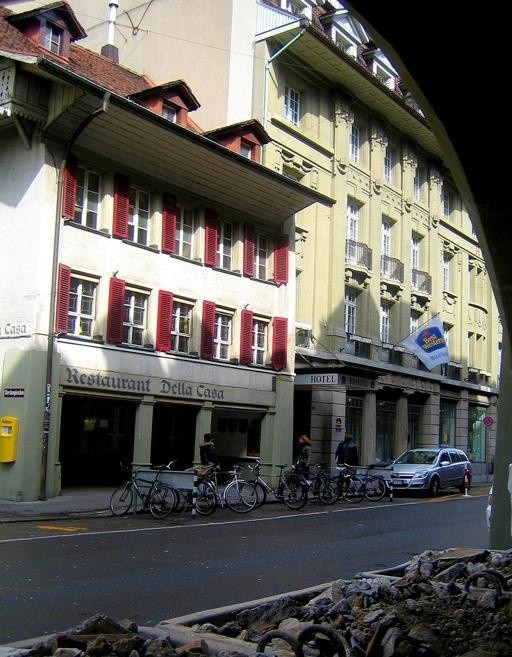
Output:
[401,315,450,371]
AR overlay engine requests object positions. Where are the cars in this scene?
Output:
[378,447,473,496]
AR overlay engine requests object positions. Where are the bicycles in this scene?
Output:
[107,456,395,518]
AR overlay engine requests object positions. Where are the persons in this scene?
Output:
[294,434,313,491]
[200,433,218,502]
[335,433,360,492]
